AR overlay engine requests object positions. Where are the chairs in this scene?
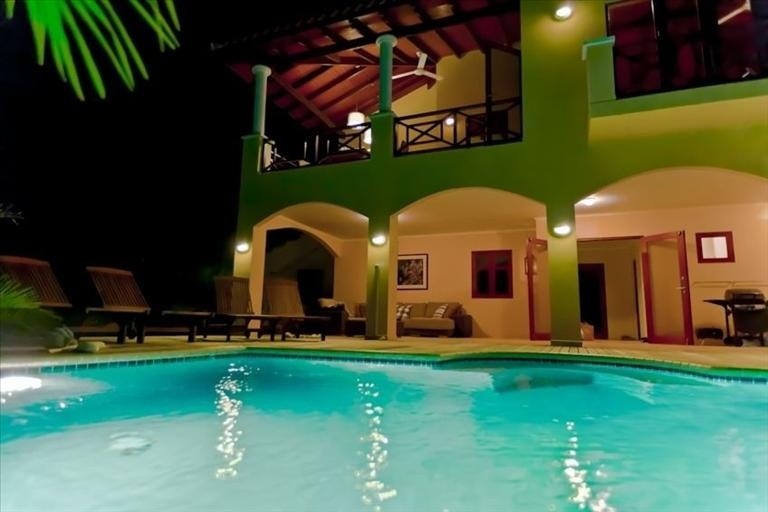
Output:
[164,276,255,342]
[86,265,151,343]
[1,256,73,317]
[215,277,329,341]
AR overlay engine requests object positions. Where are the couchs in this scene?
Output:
[396,302,463,338]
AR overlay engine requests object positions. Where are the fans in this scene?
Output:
[392,51,443,81]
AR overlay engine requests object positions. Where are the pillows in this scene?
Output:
[396,304,413,320]
[432,304,449,318]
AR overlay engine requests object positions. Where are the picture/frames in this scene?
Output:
[397,254,428,290]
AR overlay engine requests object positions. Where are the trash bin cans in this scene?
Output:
[321,304,348,336]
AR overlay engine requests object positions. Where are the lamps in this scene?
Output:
[370,233,387,247]
[584,197,595,206]
[524,255,538,275]
[363,81,375,144]
[236,243,249,253]
[553,222,571,235]
[346,64,365,129]
[554,2,572,21]
